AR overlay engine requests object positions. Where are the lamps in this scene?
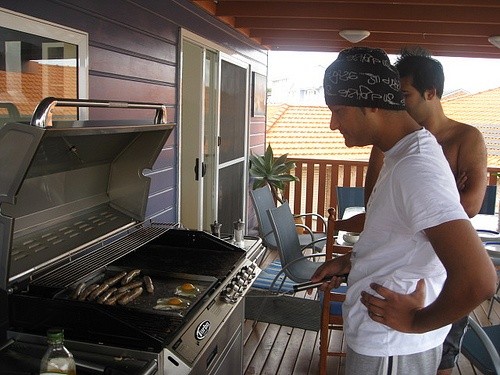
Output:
[340,30,370,44]
[488,36,500,49]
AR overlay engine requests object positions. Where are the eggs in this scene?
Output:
[174,283,206,298]
[153,296,190,311]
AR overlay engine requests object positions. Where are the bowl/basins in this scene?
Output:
[343,233,360,243]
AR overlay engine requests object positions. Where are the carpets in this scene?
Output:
[252,250,301,295]
[247,292,323,338]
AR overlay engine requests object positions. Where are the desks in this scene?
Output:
[334,204,500,295]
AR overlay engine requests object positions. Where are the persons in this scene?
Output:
[364,54,488,375]
[307,45,498,375]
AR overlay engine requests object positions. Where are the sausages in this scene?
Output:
[70,269,154,305]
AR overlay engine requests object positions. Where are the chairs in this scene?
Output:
[250,185,328,264]
[456,316,500,375]
[323,208,380,373]
[338,186,366,219]
[258,201,327,328]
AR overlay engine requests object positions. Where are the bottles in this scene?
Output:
[210,220,222,238]
[39,329,76,375]
[233,218,244,246]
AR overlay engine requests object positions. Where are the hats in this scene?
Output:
[324,46,407,111]
